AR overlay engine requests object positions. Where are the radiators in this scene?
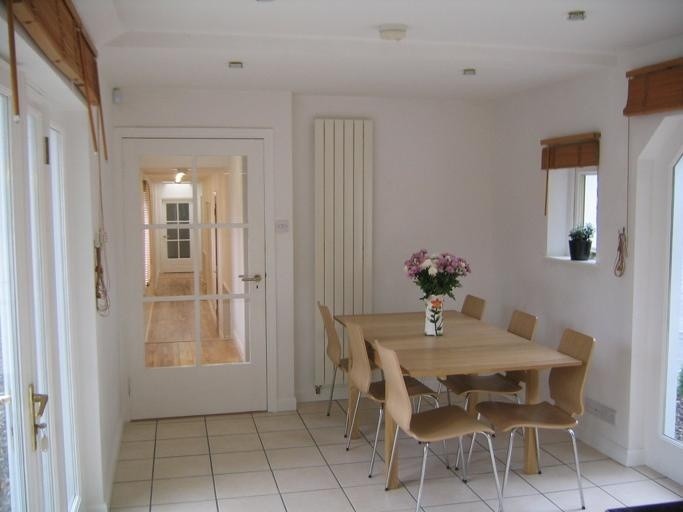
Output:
[313,119,373,394]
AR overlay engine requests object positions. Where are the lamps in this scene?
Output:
[172,168,192,183]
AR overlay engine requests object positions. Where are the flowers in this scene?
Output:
[404,247,471,301]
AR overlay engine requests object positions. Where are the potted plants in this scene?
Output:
[569,224,594,259]
